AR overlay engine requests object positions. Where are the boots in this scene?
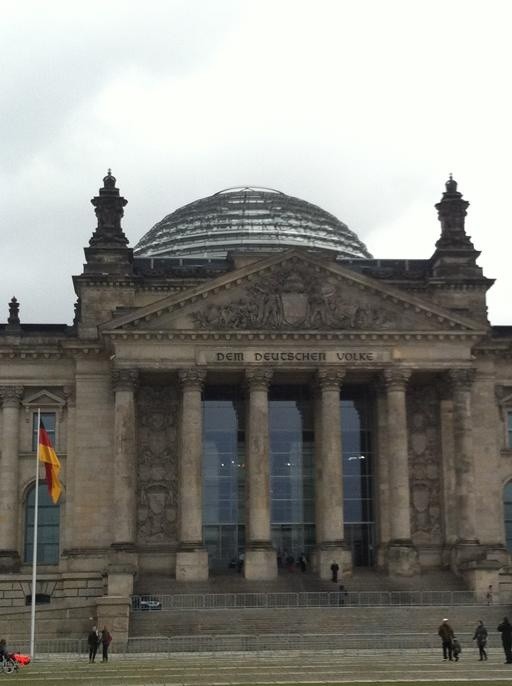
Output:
[89,657,108,663]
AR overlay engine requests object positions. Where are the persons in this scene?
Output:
[338,584,348,606]
[87,625,99,664]
[497,616,512,664]
[438,617,455,662]
[99,624,113,663]
[238,551,244,578]
[286,551,296,572]
[278,550,284,568]
[330,559,340,583]
[487,583,495,605]
[0,638,10,662]
[299,552,309,571]
[472,619,490,661]
[450,636,462,662]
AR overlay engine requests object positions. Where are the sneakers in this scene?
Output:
[504,660,512,664]
[444,657,459,661]
[478,657,488,661]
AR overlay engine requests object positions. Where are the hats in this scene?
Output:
[443,619,448,621]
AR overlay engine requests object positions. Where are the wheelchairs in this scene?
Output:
[1,651,17,674]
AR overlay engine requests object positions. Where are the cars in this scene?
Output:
[132,592,162,611]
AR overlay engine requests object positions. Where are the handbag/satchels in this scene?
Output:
[108,633,112,640]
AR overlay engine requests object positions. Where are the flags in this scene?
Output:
[38,414,63,505]
